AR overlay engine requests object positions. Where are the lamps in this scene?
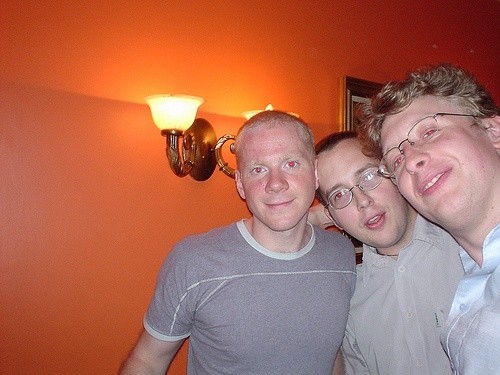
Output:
[147,92,302,182]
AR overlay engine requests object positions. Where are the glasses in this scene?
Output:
[324,166,384,210]
[376,111,486,178]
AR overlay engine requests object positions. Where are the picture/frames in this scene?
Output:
[339,72,384,265]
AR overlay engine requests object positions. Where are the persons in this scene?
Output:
[306,64,500,375]
[122,111,356,375]
[313,132,465,375]
